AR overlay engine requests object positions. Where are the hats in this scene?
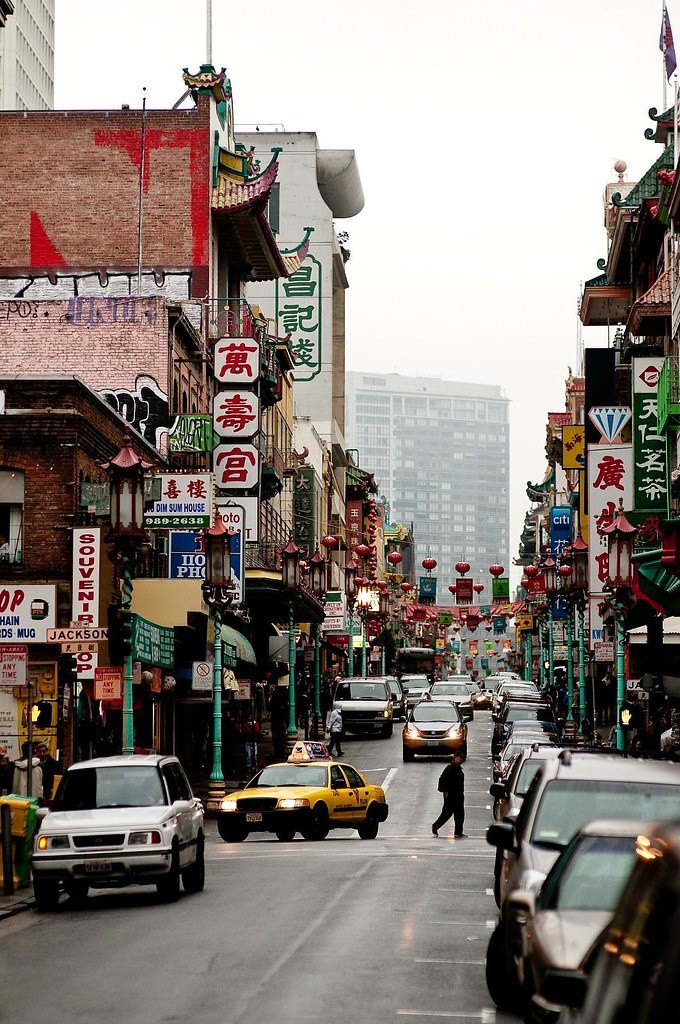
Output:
[454,751,465,756]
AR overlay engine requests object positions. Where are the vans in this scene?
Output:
[330,674,432,739]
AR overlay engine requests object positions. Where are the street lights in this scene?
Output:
[555,524,589,744]
[375,587,394,680]
[599,497,640,750]
[309,542,329,745]
[200,503,239,827]
[353,570,374,680]
[343,551,362,677]
[537,549,560,692]
[280,532,306,759]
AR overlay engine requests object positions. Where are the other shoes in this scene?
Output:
[338,752,345,755]
[329,752,334,756]
[432,824,438,835]
[454,835,467,838]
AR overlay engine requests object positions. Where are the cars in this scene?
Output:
[424,674,490,721]
[216,741,388,843]
[399,700,471,763]
[484,672,680,1024]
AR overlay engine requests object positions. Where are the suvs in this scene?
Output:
[30,754,205,912]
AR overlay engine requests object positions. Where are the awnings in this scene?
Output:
[304,630,348,658]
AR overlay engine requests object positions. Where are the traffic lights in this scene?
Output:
[32,702,53,726]
[619,701,643,731]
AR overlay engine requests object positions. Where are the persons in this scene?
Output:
[0,738,62,888]
[431,753,469,838]
[620,691,658,759]
[266,669,346,758]
[541,669,600,737]
[600,673,617,726]
[366,662,374,676]
[192,704,261,779]
[325,701,346,757]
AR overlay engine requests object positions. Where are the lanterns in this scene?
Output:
[322,535,337,550]
[455,562,470,576]
[377,579,388,591]
[392,608,520,659]
[401,582,411,593]
[387,551,402,568]
[489,563,505,578]
[473,583,484,594]
[449,585,457,595]
[521,578,529,588]
[523,564,540,578]
[353,577,364,588]
[355,543,369,560]
[422,557,437,572]
[558,564,573,583]
